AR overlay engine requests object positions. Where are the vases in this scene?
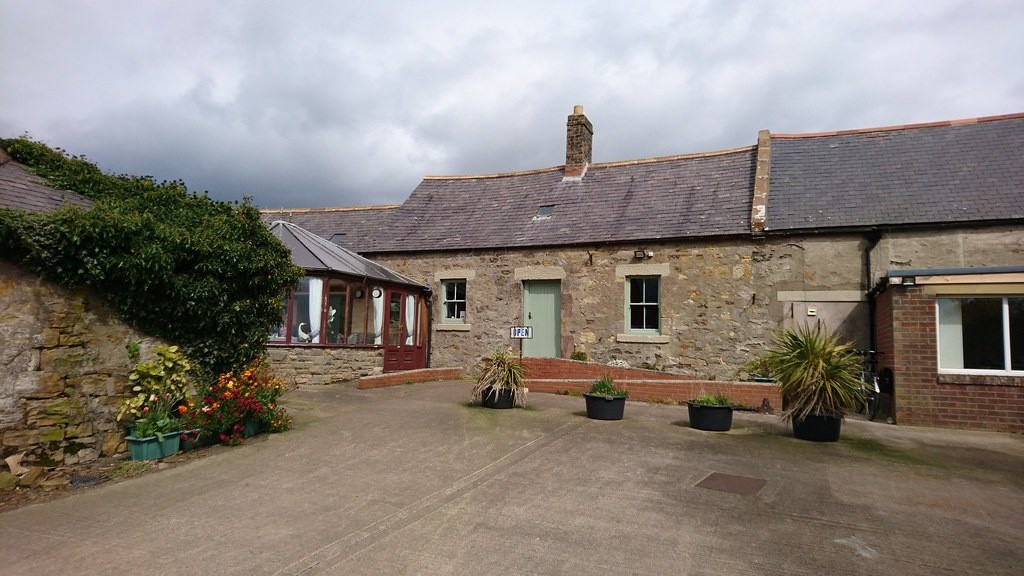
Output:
[123,430,183,462]
[179,429,210,451]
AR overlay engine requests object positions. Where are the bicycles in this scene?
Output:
[845,348,885,421]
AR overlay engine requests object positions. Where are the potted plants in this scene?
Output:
[469,343,530,410]
[756,318,877,442]
[684,374,735,432]
[583,369,630,420]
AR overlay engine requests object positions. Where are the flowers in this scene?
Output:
[115,343,299,446]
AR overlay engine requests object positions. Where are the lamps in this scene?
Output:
[634,250,646,259]
